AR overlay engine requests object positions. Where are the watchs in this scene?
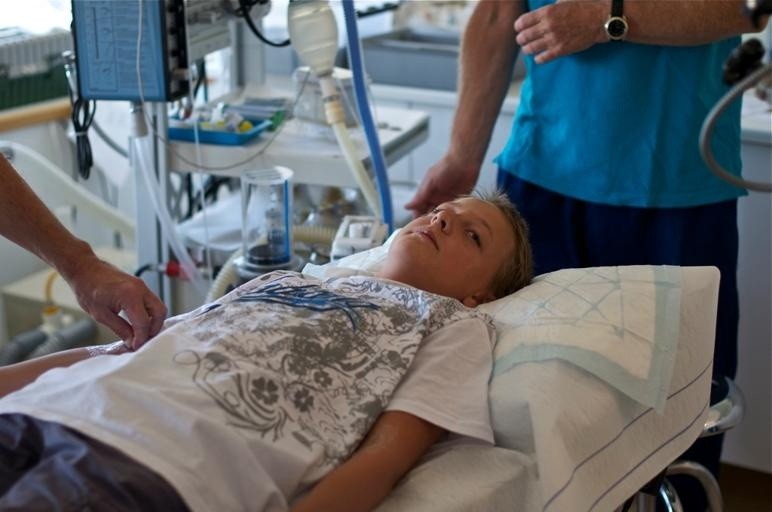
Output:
[604,0,629,43]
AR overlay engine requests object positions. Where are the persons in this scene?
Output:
[0,175,535,510]
[406,0,770,512]
[0,149,170,350]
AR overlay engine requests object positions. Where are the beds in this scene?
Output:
[301,228,745,511]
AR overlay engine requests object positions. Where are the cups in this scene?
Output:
[241,164,296,265]
